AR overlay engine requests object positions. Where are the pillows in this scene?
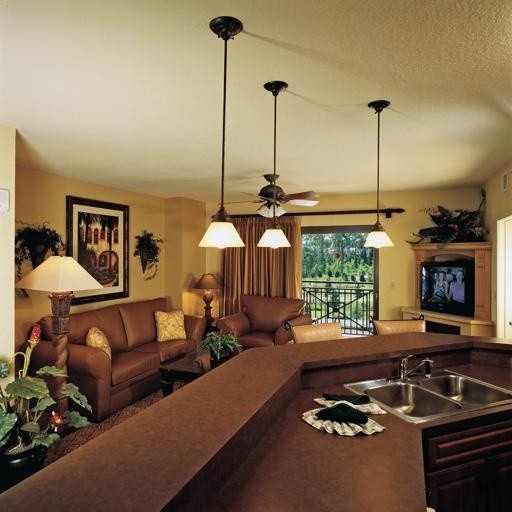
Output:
[86,327,112,361]
[154,309,188,342]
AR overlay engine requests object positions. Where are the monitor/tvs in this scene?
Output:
[420,260,474,317]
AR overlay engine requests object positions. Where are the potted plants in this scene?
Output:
[198,331,243,368]
[133,229,163,281]
[0,323,96,478]
[15,217,66,299]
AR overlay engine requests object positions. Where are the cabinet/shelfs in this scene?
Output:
[425,418,512,512]
[412,242,493,321]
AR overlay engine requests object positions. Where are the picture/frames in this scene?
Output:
[65,194,130,305]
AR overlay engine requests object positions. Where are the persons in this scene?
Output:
[427,270,449,303]
[448,270,466,304]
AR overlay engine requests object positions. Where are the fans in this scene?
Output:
[215,173,323,210]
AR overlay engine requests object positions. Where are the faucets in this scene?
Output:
[398,354,425,381]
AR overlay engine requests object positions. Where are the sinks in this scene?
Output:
[344,380,464,426]
[403,369,512,421]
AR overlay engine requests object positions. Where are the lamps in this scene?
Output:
[256,80,295,250]
[15,244,103,418]
[362,99,395,249]
[191,273,223,324]
[197,15,247,251]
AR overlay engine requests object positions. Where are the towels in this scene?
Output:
[301,393,389,437]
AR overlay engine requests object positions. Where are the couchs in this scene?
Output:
[15,296,207,423]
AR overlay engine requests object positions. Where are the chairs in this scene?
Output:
[216,294,426,349]
